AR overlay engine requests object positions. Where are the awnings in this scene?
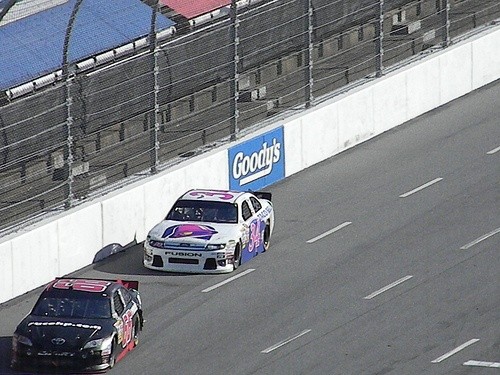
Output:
[0,0,178,102]
[158,1,289,28]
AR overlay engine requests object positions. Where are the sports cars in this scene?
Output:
[142,188,275,274]
[8,276,144,375]
